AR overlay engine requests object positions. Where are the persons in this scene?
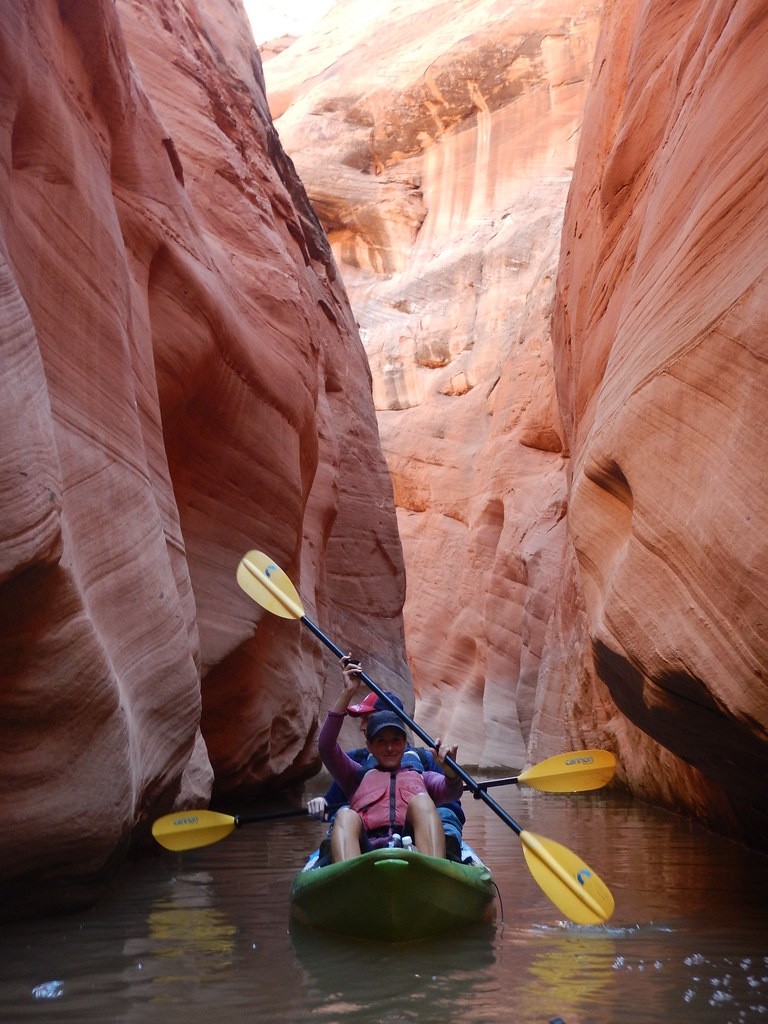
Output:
[317,653,463,863]
[307,690,466,868]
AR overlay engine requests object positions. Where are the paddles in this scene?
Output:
[150,747,618,854]
[232,544,619,931]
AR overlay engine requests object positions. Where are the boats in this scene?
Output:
[288,828,494,941]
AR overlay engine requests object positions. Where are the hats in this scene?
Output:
[347,690,403,718]
[365,710,407,739]
